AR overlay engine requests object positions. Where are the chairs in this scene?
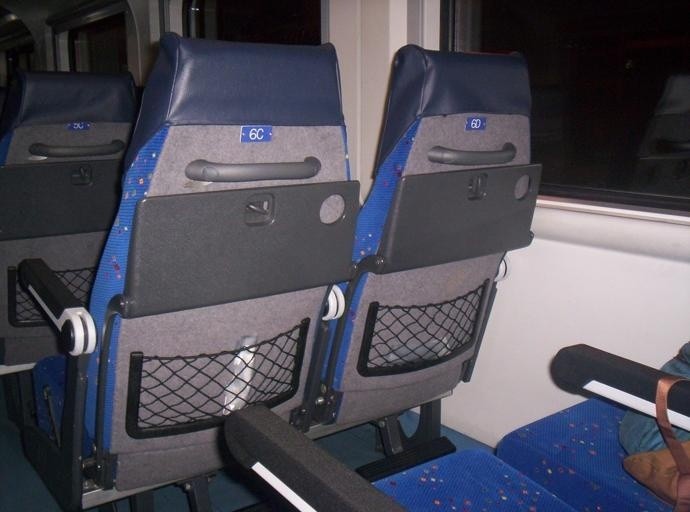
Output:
[224,404,581,512]
[0,72,138,431]
[312,44,544,486]
[16,31,360,512]
[495,344,690,510]
